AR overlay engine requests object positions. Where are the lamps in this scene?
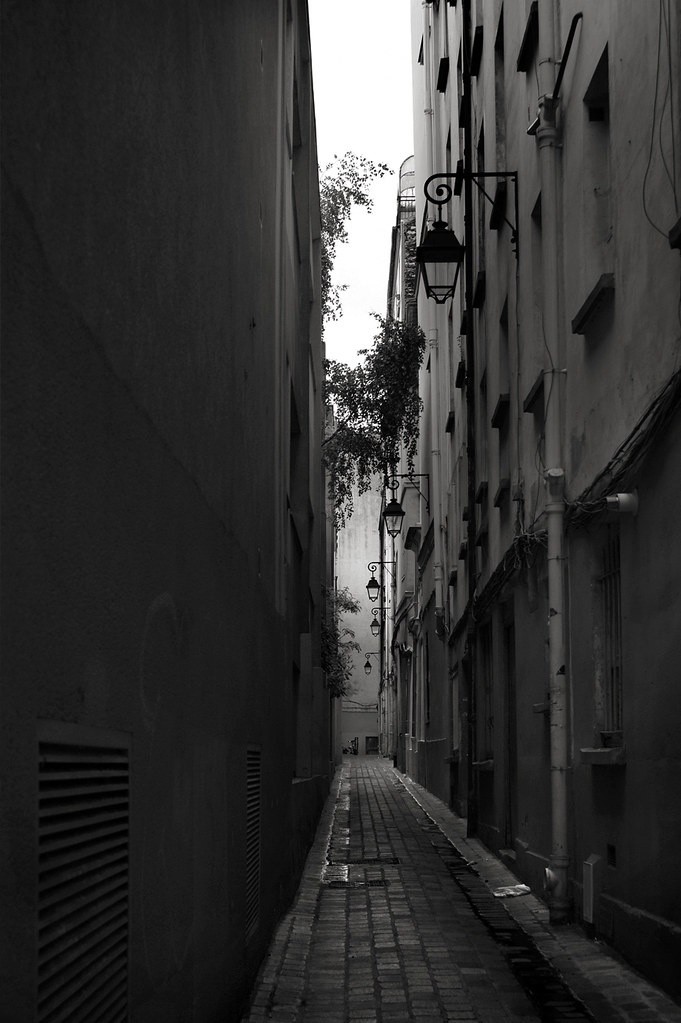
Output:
[366,562,396,602]
[370,608,391,637]
[415,171,518,304]
[364,652,380,674]
[382,474,430,538]
